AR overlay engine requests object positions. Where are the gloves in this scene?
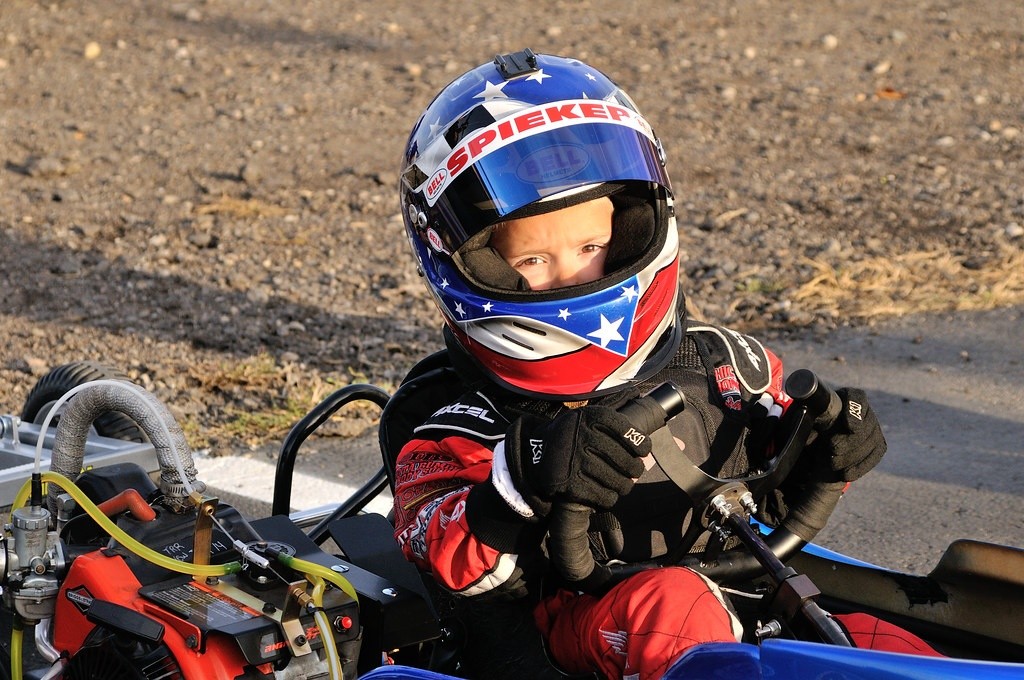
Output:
[777,386,887,483]
[505,386,652,517]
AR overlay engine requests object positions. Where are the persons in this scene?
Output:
[380,48,949,680]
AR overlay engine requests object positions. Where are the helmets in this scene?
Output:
[400,49,680,402]
